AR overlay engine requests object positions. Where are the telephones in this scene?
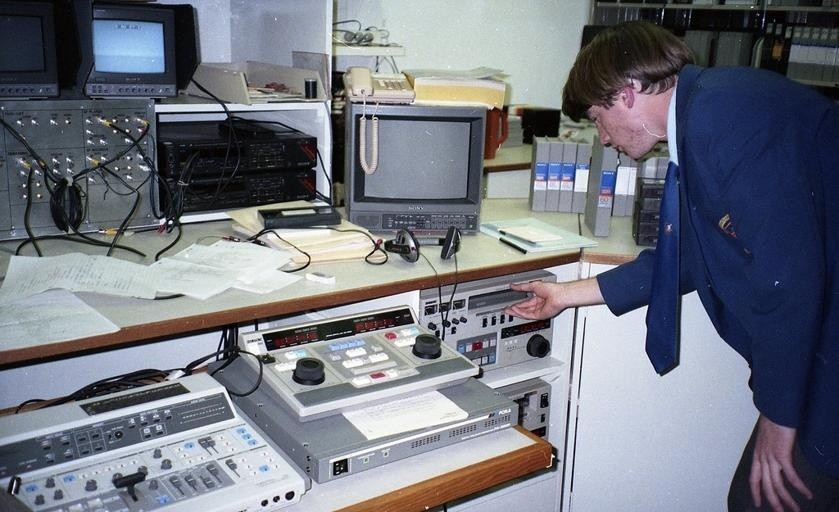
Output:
[343,66,415,104]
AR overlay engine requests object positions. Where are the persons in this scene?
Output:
[504,19,837,511]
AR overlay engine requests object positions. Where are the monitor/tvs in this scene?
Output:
[83,2,198,100]
[0,0,61,100]
[346,104,487,236]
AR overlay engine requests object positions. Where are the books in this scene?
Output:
[481,214,598,253]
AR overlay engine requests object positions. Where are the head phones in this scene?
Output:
[384,226,465,264]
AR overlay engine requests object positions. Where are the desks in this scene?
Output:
[0,200,755,511]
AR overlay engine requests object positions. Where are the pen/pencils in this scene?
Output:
[500,237,527,254]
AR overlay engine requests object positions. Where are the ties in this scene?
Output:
[644,158,682,376]
